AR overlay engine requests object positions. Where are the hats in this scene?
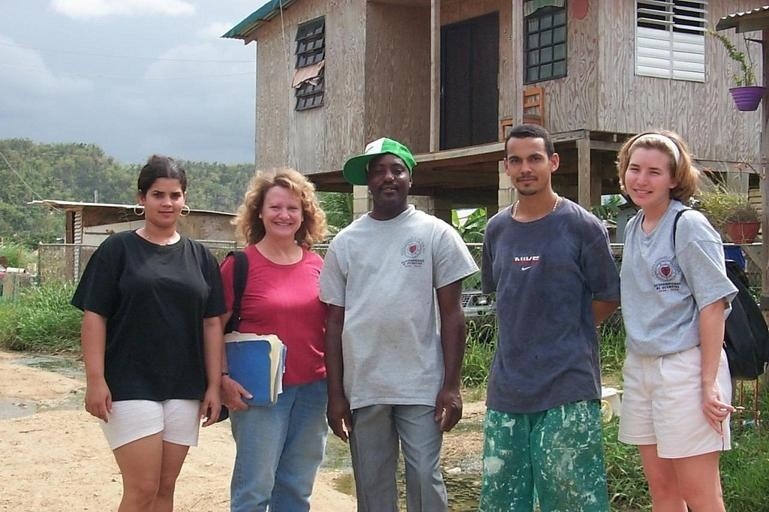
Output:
[342,137,418,186]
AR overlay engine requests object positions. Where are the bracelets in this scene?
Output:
[220,371,230,377]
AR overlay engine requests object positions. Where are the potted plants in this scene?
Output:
[700,28,766,114]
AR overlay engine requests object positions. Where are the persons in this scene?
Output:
[478,123,623,512]
[68,154,228,511]
[613,128,738,511]
[216,164,330,512]
[315,138,482,511]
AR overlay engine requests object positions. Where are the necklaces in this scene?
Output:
[512,192,560,219]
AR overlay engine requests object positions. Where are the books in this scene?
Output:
[225,328,288,408]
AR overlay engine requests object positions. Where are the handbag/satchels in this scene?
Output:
[671,209,769,380]
[206,250,249,423]
